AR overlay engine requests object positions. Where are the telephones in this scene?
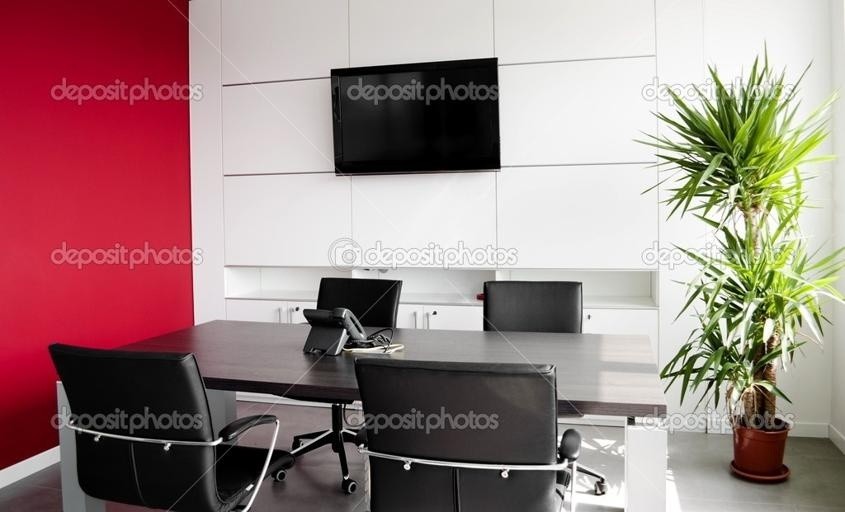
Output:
[303,307,368,356]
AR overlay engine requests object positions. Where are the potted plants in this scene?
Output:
[630,35,844,485]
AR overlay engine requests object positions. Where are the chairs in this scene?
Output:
[350,350,580,511]
[285,275,406,494]
[477,279,606,497]
[46,341,295,512]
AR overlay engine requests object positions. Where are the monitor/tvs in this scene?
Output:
[329,57,501,177]
